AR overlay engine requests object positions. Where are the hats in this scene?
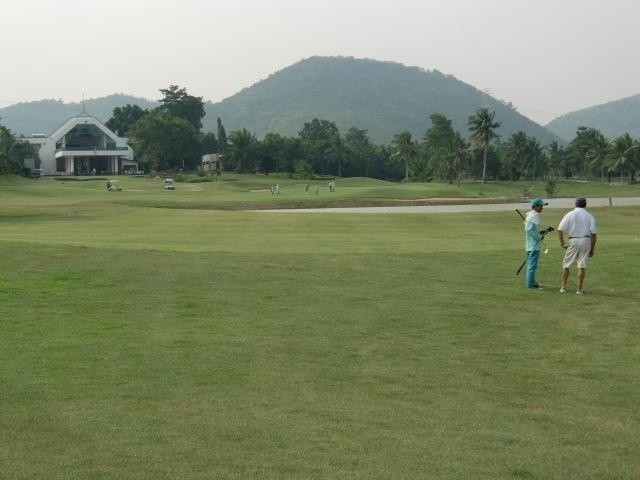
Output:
[575,196,586,207]
[531,198,548,207]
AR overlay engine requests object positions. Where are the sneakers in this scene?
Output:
[559,288,565,292]
[529,283,543,290]
[577,291,585,295]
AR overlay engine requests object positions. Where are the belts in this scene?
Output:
[569,235,591,239]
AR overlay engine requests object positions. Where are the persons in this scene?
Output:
[304,183,310,195]
[523,198,549,289]
[274,184,280,197]
[332,181,336,193]
[270,184,275,197]
[328,182,333,192]
[559,196,598,295]
[315,186,319,195]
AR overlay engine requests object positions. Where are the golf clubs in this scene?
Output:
[517,227,554,274]
[516,208,548,254]
